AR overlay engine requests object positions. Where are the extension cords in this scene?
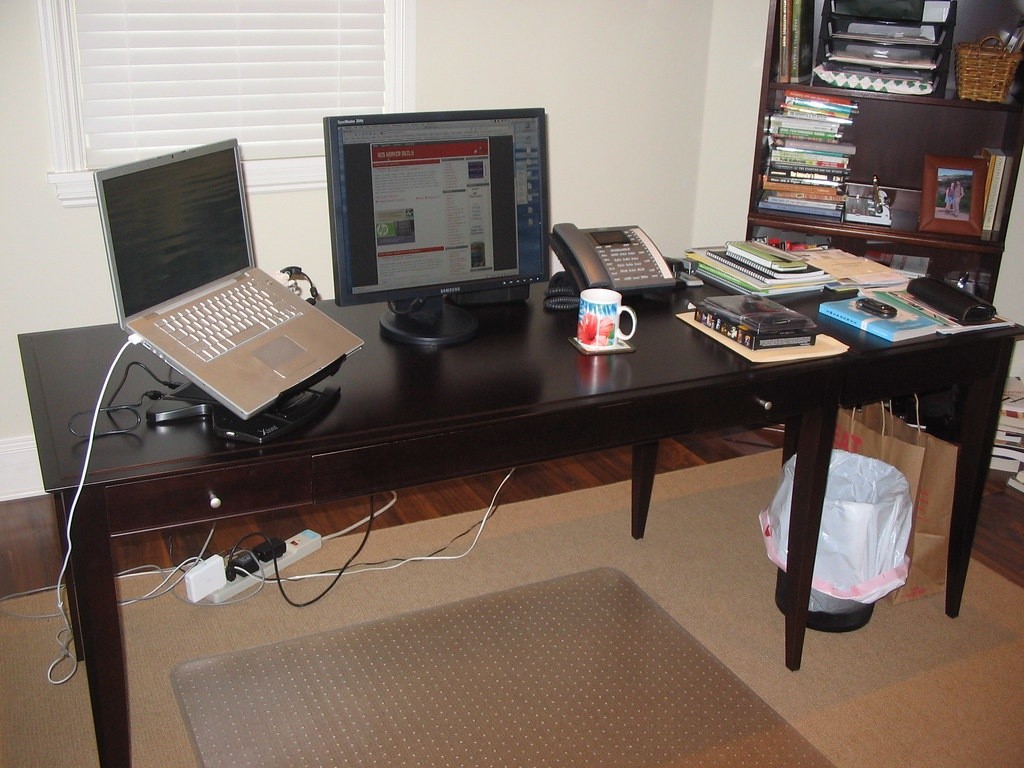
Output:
[205,529,322,603]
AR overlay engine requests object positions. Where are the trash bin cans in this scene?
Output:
[776,450,908,633]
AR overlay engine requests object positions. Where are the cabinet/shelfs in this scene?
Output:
[744,0,1024,308]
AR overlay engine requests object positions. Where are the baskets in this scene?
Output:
[955,28,1023,103]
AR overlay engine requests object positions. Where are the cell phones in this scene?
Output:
[856,298,896,318]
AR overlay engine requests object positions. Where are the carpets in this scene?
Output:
[168,566,846,768]
[0,447,1024,768]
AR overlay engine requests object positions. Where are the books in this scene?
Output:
[981,147,1015,242]
[777,0,812,83]
[675,226,1015,363]
[987,378,1024,494]
[758,90,892,226]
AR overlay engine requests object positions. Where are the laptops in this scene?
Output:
[92,137,365,419]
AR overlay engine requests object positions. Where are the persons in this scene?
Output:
[944,180,965,216]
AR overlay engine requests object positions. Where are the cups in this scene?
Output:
[577,289,637,348]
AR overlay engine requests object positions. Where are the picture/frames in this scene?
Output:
[918,152,987,240]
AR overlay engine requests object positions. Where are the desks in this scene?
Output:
[18,242,1024,768]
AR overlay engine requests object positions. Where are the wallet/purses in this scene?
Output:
[906,276,998,324]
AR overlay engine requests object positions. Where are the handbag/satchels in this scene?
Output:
[832,394,958,605]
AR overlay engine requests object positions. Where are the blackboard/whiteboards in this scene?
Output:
[550,223,677,294]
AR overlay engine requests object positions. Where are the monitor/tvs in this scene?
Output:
[324,107,550,344]
